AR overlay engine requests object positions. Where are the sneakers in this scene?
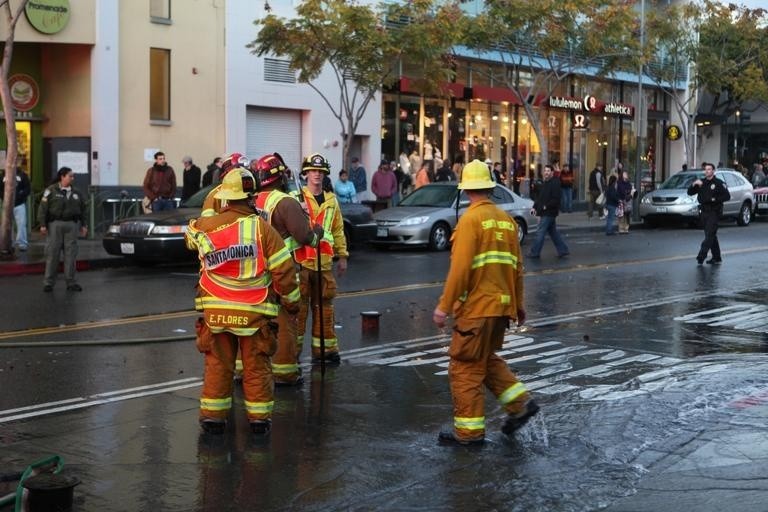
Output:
[200,419,227,430]
[66,285,82,292]
[500,400,541,435]
[18,244,27,249]
[554,250,570,258]
[697,255,723,264]
[43,286,52,291]
[250,419,272,440]
[438,430,458,441]
[524,251,540,258]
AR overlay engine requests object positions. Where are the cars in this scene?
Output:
[372,182,536,249]
[641,167,768,226]
[104,181,377,263]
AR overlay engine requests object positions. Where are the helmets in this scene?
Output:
[456,159,496,190]
[301,153,331,175]
[212,153,287,203]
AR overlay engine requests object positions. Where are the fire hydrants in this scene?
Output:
[22,470,81,510]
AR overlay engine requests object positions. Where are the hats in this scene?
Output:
[181,156,193,162]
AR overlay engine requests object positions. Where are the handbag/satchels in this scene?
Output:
[141,196,152,213]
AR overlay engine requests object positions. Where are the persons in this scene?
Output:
[183,167,301,435]
[423,139,433,160]
[735,161,767,201]
[588,163,632,235]
[37,166,87,290]
[432,158,541,446]
[231,153,324,386]
[288,152,348,363]
[682,164,687,170]
[526,165,570,258]
[323,158,503,210]
[687,162,730,264]
[143,153,176,214]
[183,158,260,203]
[1,159,29,252]
[511,161,573,212]
[471,136,479,157]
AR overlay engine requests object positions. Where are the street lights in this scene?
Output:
[733,107,741,165]
